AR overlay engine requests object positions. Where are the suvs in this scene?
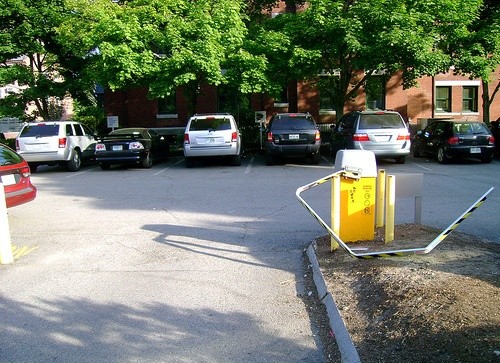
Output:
[329,110,412,164]
[265,112,321,165]
[183,113,242,166]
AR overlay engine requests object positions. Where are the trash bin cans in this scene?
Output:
[331,148,377,244]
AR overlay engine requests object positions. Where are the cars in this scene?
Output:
[95,128,168,168]
[15,121,98,172]
[0,142,37,208]
[412,120,497,164]
